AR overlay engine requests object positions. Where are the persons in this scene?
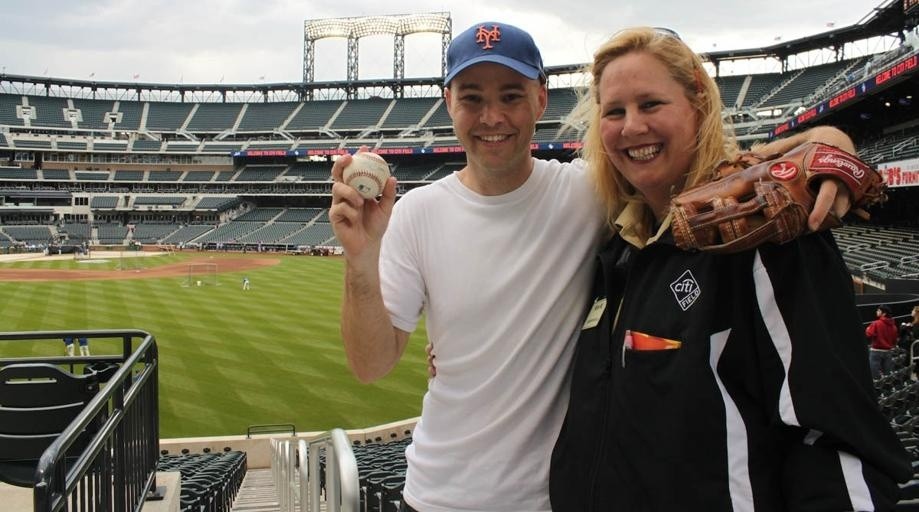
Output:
[900,306,919,351]
[327,19,854,512]
[242,277,250,291]
[63,338,77,356]
[864,306,898,380]
[548,19,914,512]
[77,338,90,356]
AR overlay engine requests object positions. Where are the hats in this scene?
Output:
[443,22,549,86]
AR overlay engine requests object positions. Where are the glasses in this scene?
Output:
[610,27,702,94]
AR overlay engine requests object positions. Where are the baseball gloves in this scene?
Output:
[671,142,889,259]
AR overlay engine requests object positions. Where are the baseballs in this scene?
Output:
[343,152,389,200]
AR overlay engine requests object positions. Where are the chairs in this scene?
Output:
[157,450,247,512]
[296,436,412,512]
[873,363,919,512]
[0,363,110,488]
